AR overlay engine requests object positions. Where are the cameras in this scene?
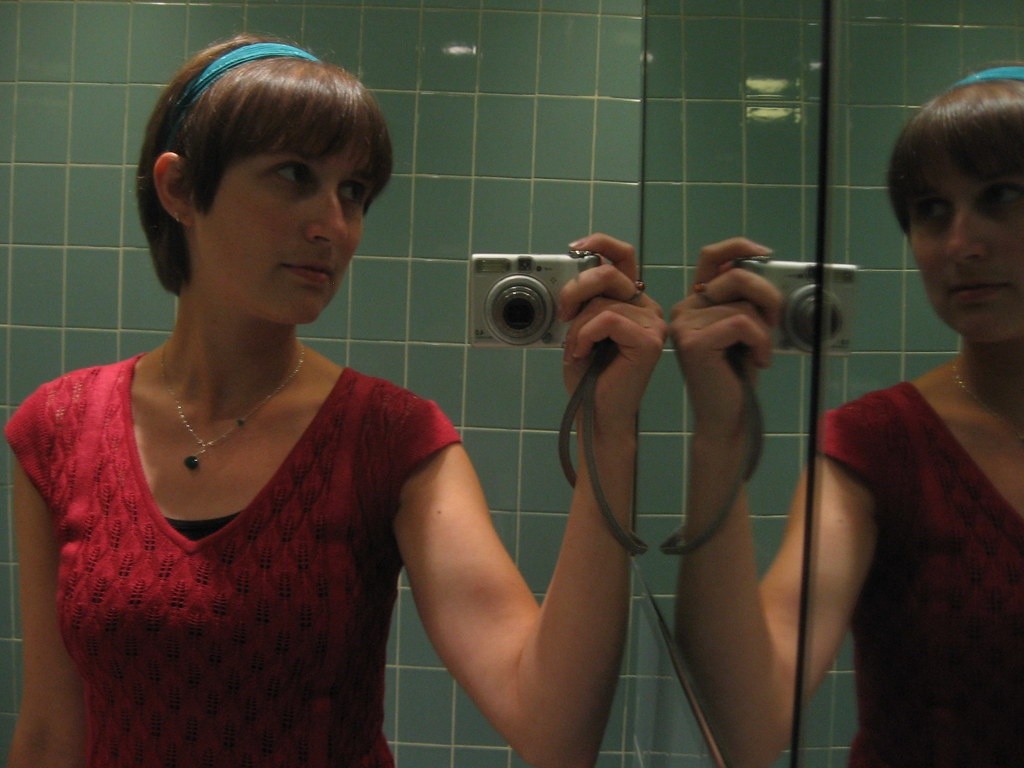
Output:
[732,255,858,354]
[469,247,604,349]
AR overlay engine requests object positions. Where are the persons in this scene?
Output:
[3,32,671,768]
[672,61,1024,768]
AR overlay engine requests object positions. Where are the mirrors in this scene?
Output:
[634,0,1024,768]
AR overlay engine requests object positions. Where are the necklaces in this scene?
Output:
[951,358,1024,439]
[160,333,304,470]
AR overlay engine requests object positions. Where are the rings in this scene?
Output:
[692,279,714,304]
[630,280,645,302]
